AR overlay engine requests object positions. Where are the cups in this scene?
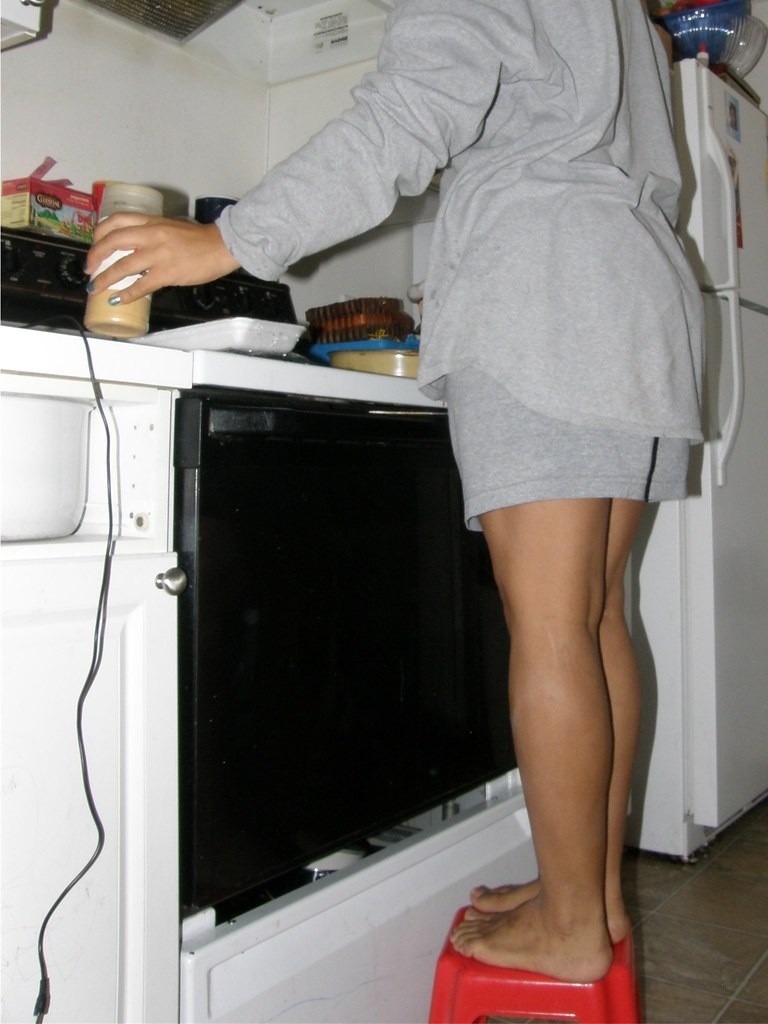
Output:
[83,183,165,340]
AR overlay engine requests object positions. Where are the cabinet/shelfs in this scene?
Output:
[0,372,187,1024]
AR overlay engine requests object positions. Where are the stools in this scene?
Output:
[429,906,641,1024]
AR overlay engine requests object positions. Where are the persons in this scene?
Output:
[85,0,705,983]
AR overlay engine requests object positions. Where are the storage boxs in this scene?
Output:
[1,177,96,247]
